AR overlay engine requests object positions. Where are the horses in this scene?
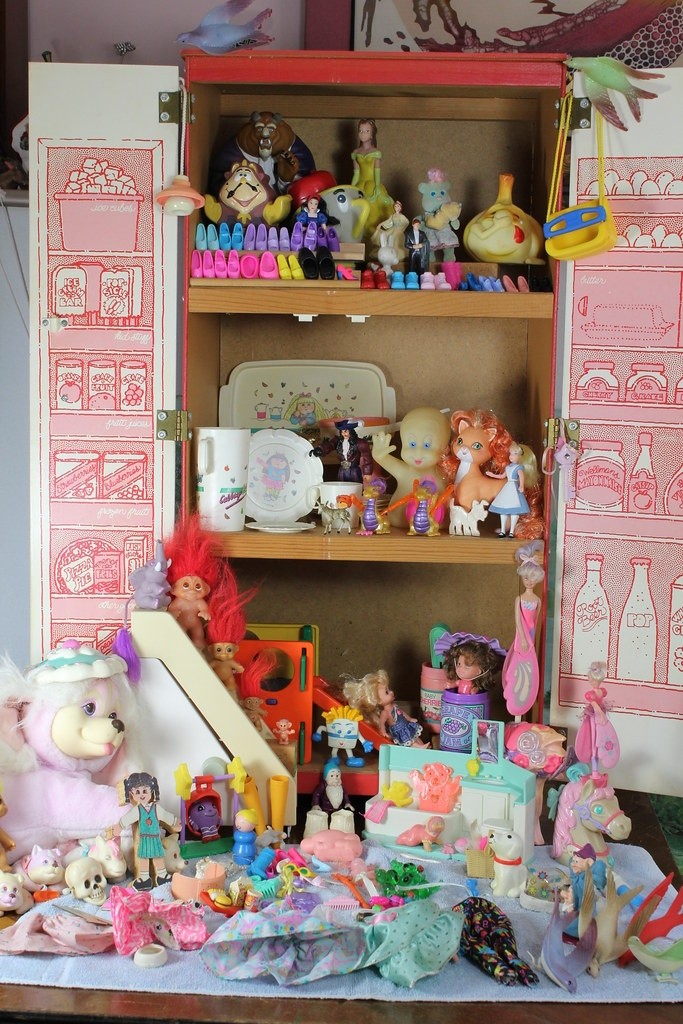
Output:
[552,762,632,871]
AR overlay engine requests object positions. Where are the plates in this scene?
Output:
[244,430,323,522]
[243,521,316,534]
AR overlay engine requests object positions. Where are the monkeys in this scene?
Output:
[273,719,296,744]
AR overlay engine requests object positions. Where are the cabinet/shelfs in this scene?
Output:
[167,48,562,834]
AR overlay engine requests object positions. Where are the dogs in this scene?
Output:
[488,831,528,898]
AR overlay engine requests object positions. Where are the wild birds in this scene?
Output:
[522,861,683,996]
[173,1,275,55]
[562,57,666,131]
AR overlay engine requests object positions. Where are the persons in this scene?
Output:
[485,445,530,539]
[561,843,607,917]
[404,219,429,274]
[310,757,355,813]
[351,119,395,235]
[371,200,409,260]
[313,420,373,483]
[296,193,328,231]
[574,662,620,778]
[343,670,431,749]
[502,541,546,723]
[165,517,276,732]
[434,631,508,695]
[371,407,450,532]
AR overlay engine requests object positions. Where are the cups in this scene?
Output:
[303,810,328,838]
[331,809,354,835]
[134,944,167,967]
[440,688,489,754]
[419,664,453,735]
[307,481,364,528]
[192,427,249,533]
